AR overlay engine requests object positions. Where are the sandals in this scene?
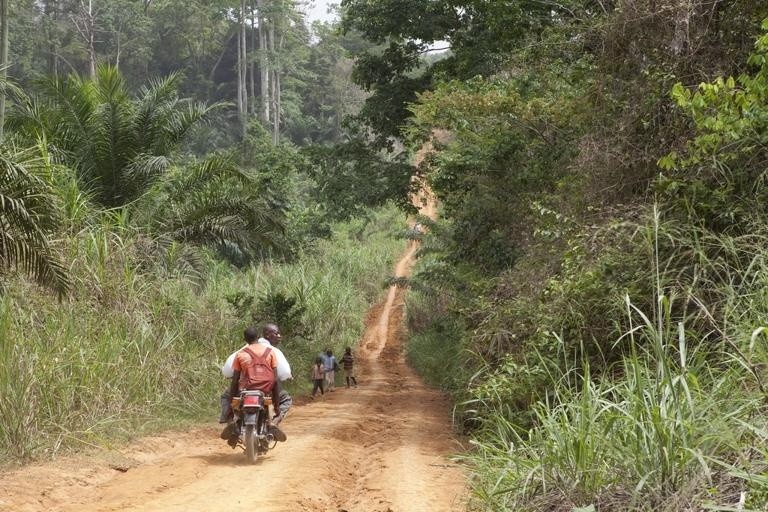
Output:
[269,425,286,442]
[221,422,236,439]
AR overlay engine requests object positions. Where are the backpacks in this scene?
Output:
[244,346,276,394]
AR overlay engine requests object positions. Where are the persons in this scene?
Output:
[307,357,326,399]
[319,347,340,394]
[337,345,359,388]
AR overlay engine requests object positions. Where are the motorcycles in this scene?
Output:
[225,376,294,464]
